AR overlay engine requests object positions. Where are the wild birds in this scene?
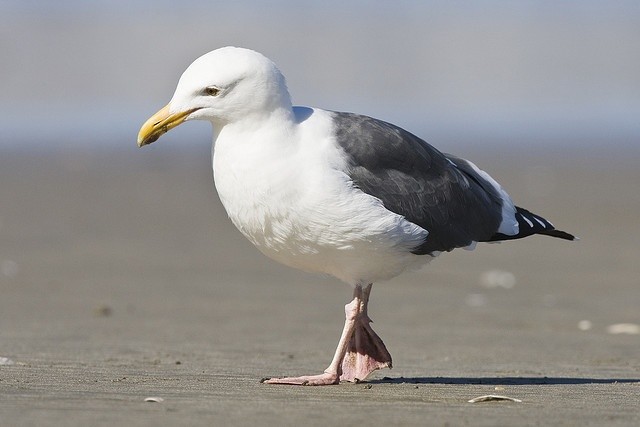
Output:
[136,43,582,386]
